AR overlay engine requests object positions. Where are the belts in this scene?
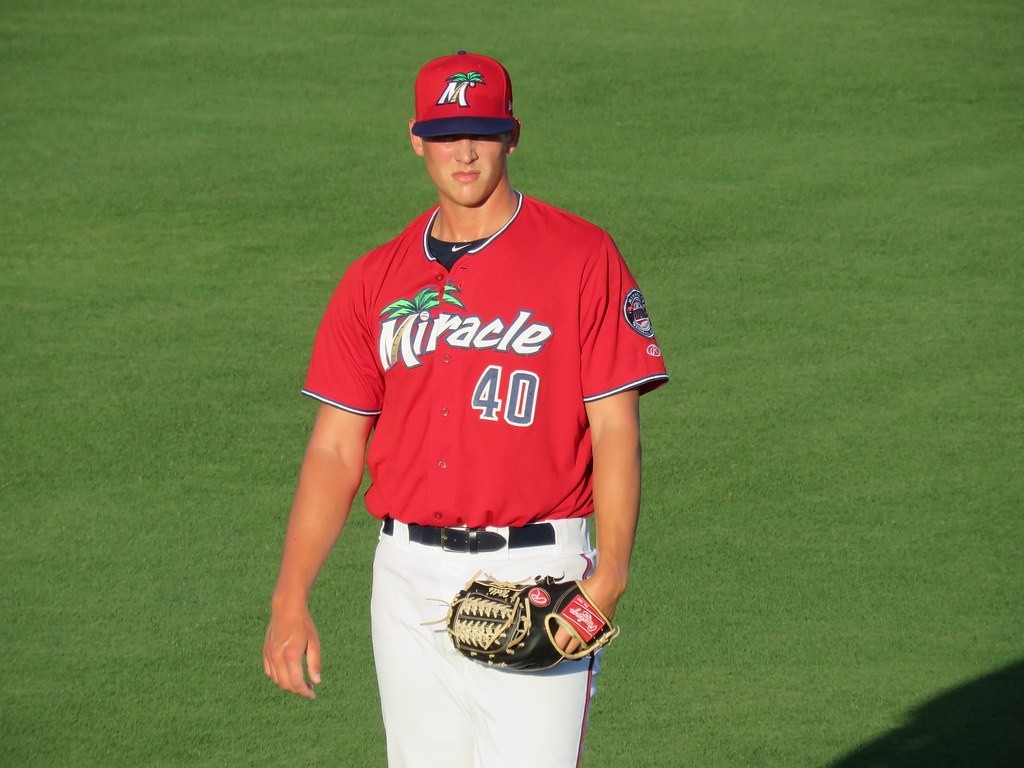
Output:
[381,515,559,555]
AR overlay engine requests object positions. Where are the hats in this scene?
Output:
[411,50,515,137]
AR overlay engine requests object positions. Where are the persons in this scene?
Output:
[261,50,669,768]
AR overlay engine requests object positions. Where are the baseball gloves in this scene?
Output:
[446,574,621,674]
